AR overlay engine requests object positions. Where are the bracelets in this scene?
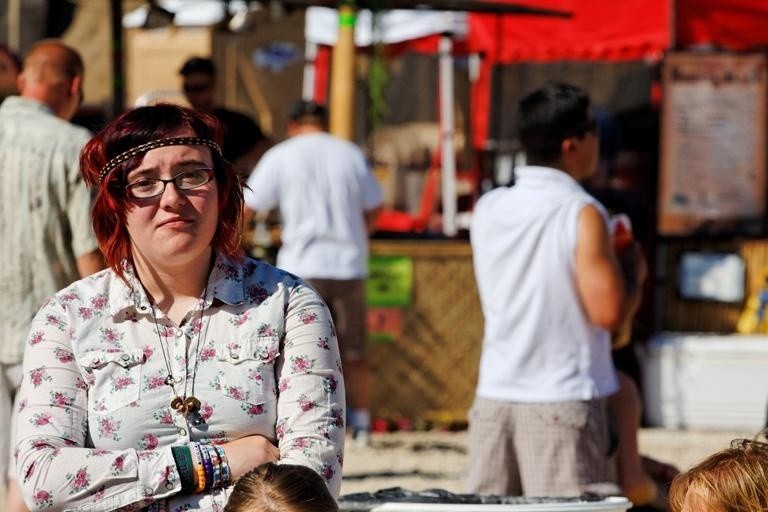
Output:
[171,440,231,495]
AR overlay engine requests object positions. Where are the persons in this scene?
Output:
[0,52,23,102]
[669,437,768,510]
[464,77,637,494]
[1,39,105,510]
[224,462,342,512]
[240,103,384,373]
[176,55,269,176]
[10,102,348,512]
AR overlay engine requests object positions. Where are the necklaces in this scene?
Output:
[149,276,208,416]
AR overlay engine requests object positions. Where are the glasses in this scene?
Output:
[123,167,216,200]
